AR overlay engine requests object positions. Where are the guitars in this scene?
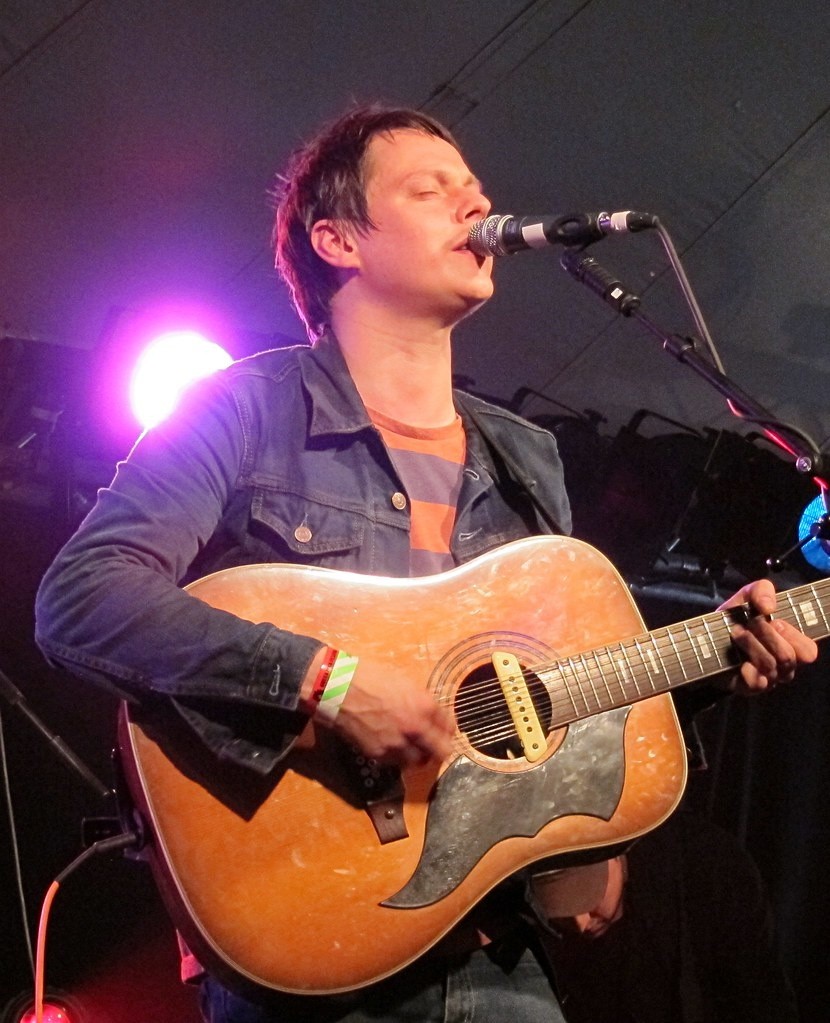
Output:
[113,527,828,1009]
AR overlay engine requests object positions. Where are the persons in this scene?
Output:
[36,95,817,1023]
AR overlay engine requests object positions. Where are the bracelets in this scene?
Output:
[306,646,340,718]
[312,649,359,730]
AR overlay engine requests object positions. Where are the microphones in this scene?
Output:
[559,248,648,314]
[468,212,658,258]
